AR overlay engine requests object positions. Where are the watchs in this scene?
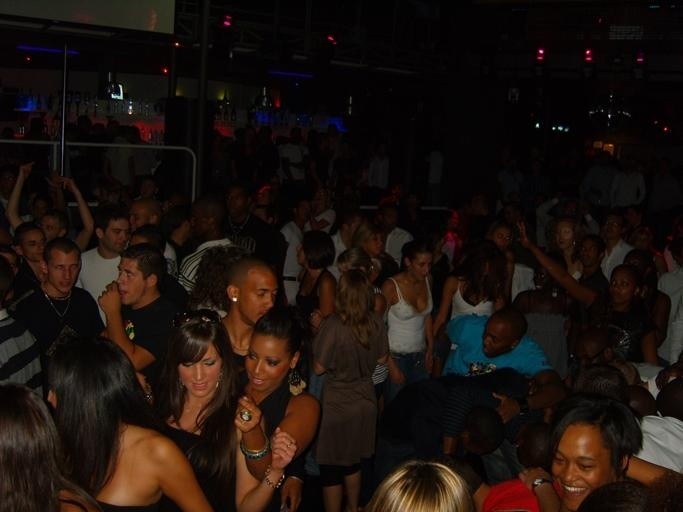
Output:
[530,478,552,496]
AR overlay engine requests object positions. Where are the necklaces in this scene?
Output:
[42,283,72,317]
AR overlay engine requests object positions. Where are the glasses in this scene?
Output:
[171,309,222,329]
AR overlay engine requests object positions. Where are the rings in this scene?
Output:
[239,411,252,421]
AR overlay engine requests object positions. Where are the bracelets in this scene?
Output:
[623,454,632,473]
[263,465,286,489]
[236,433,270,461]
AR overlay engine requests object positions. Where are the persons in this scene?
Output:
[435,307,568,422]
[328,211,361,280]
[310,269,389,512]
[519,466,653,511]
[436,241,506,334]
[178,197,231,292]
[139,175,160,199]
[492,225,513,297]
[218,257,308,511]
[622,247,672,346]
[602,216,633,280]
[538,196,578,225]
[10,176,64,235]
[274,198,310,303]
[74,206,131,326]
[578,199,607,233]
[517,218,657,363]
[165,207,190,279]
[555,219,582,278]
[575,362,683,473]
[48,335,214,512]
[220,182,272,256]
[293,230,337,317]
[97,242,180,402]
[365,461,474,512]
[5,160,93,252]
[390,368,530,456]
[232,306,320,482]
[337,246,388,400]
[150,309,297,512]
[12,221,46,299]
[187,245,246,317]
[130,199,178,275]
[375,203,413,266]
[573,230,604,284]
[483,393,682,512]
[609,156,646,209]
[130,224,177,277]
[304,186,335,234]
[14,237,104,354]
[565,328,623,387]
[0,381,96,511]
[353,221,399,287]
[381,239,436,463]
[0,255,45,399]
